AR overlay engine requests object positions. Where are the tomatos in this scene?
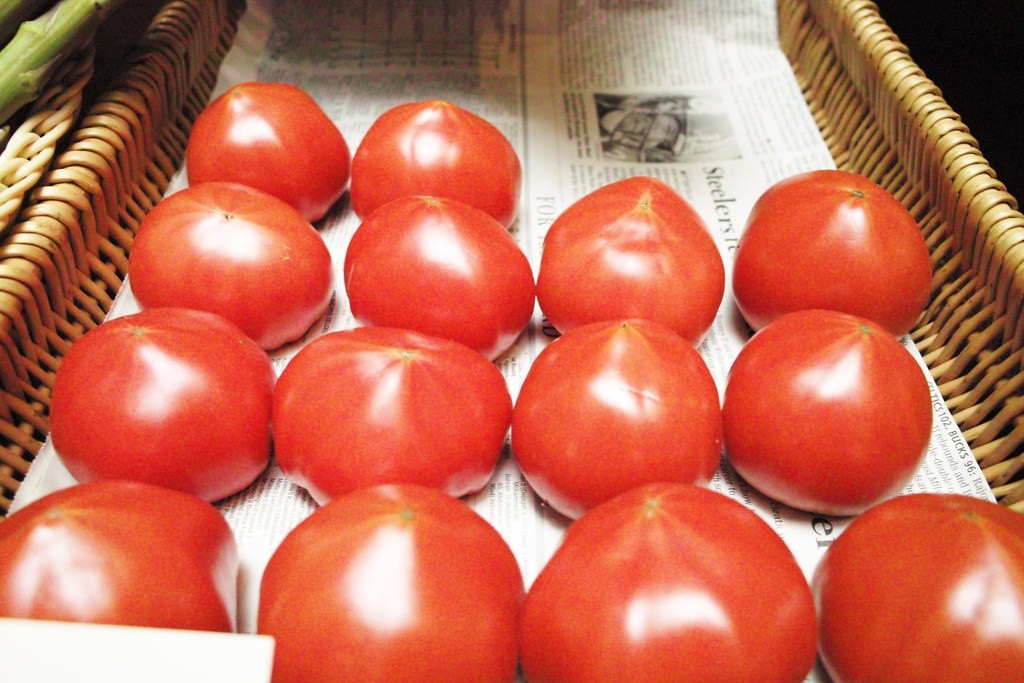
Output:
[0,80,1024,683]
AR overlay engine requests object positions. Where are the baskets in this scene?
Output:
[0,0,1024,516]
